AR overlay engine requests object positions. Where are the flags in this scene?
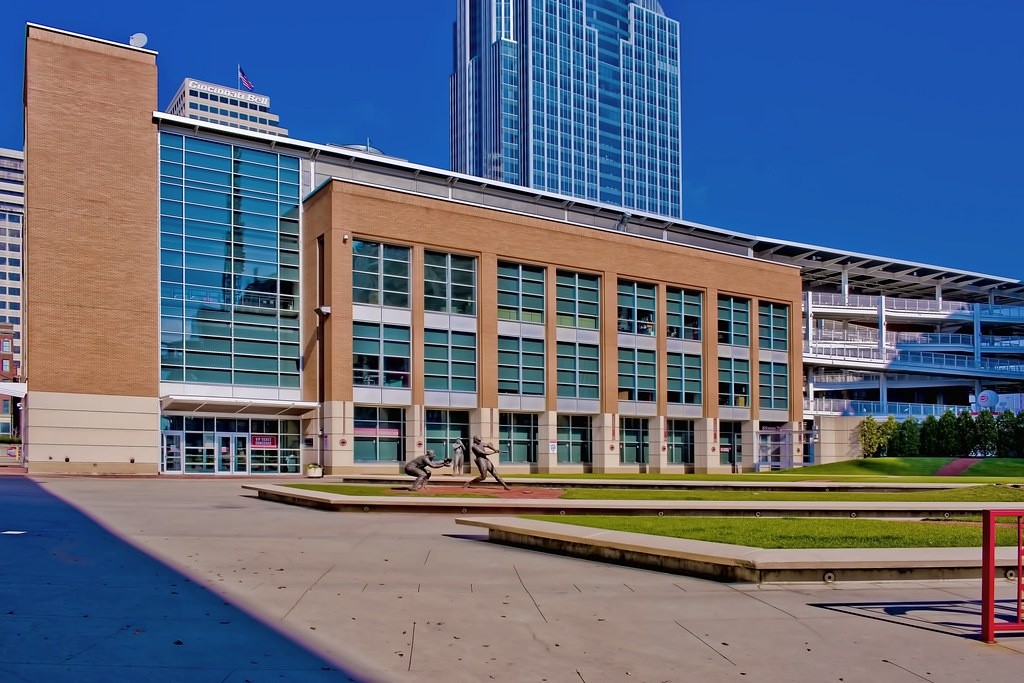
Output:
[239,68,254,90]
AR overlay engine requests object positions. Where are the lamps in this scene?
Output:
[314,306,331,317]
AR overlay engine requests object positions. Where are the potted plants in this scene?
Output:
[304,464,323,478]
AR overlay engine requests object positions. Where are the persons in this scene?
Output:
[405,450,451,491]
[463,434,511,490]
[452,438,466,476]
